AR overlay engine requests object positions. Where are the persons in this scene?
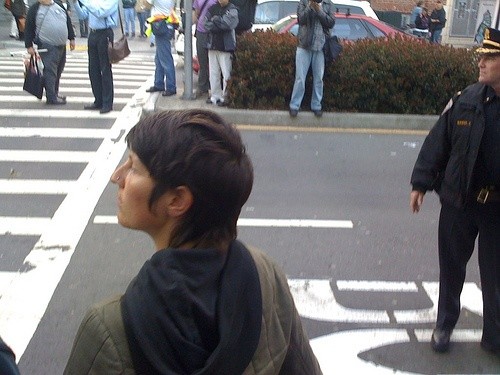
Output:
[191,0,257,106]
[63,109,323,375]
[73,0,118,113]
[24,0,75,105]
[411,27,500,354]
[9,0,31,40]
[122,0,136,36]
[60,0,89,37]
[410,1,446,44]
[290,0,336,117]
[136,0,184,47]
[145,0,177,96]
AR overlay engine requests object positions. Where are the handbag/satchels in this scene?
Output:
[323,35,342,61]
[108,34,130,64]
[151,21,167,35]
[23,53,43,99]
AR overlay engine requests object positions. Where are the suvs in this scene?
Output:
[173,0,380,62]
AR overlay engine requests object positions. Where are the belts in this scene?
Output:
[467,189,500,205]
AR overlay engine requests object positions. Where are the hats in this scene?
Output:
[474,27,500,56]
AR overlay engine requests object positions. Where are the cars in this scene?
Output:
[191,13,429,76]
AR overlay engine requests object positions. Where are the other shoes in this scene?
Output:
[432,328,450,353]
[206,99,229,107]
[311,110,322,117]
[84,103,102,110]
[100,107,112,113]
[46,96,66,106]
[162,91,176,96]
[480,339,500,356]
[146,86,165,92]
[290,109,297,117]
